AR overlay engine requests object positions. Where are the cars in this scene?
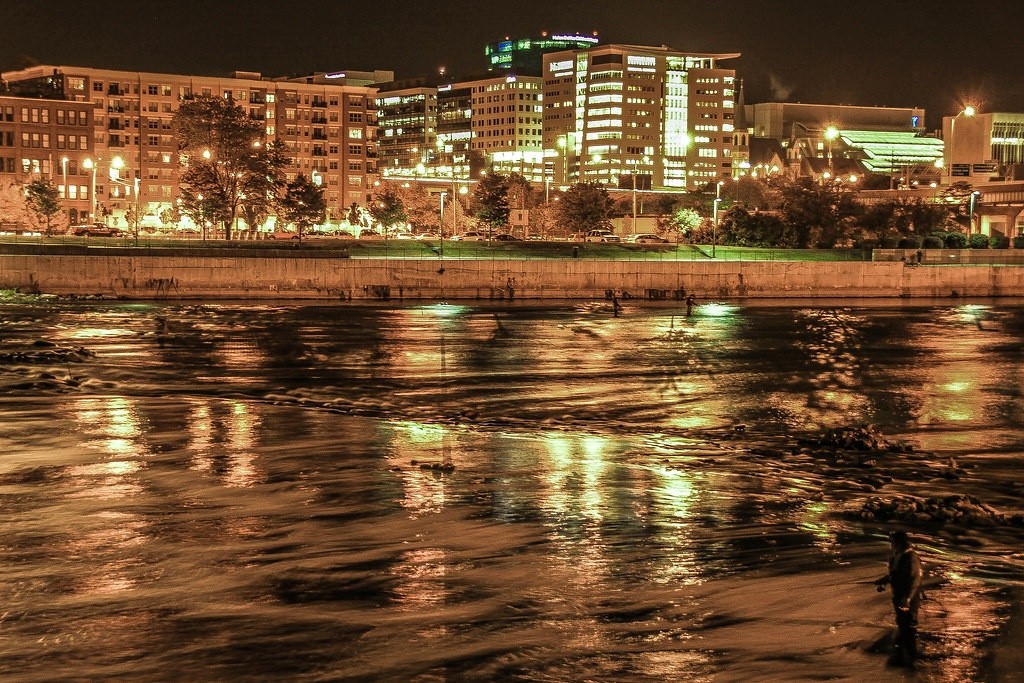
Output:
[305,230,355,239]
[492,234,523,242]
[269,230,299,239]
[416,232,439,241]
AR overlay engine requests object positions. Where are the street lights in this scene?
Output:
[970,192,980,247]
[84,158,96,225]
[113,157,139,249]
[949,106,973,186]
[712,181,724,258]
[827,128,836,181]
[440,192,448,261]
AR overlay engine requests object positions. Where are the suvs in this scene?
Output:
[627,233,668,244]
[450,232,485,242]
[586,230,620,244]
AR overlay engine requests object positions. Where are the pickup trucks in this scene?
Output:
[69,222,123,237]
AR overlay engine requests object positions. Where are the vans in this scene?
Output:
[359,229,382,239]
